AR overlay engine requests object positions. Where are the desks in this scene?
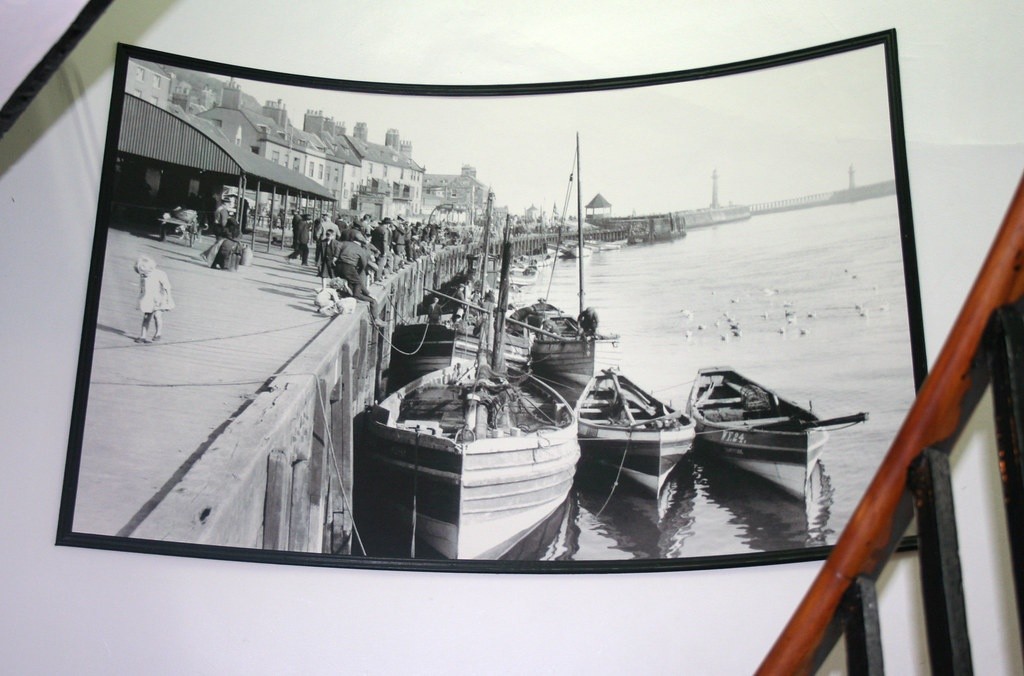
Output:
[159,218,198,247]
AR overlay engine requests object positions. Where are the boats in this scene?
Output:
[574,478,704,559]
[575,364,699,501]
[684,364,828,505]
[360,213,583,561]
[386,187,622,399]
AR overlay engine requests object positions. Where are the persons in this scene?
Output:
[312,212,475,327]
[214,197,232,241]
[135,257,174,346]
[285,210,312,266]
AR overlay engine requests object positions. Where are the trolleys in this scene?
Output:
[156,210,199,249]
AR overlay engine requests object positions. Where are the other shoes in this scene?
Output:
[151,336,161,341]
[370,317,388,327]
[134,337,146,343]
[200,253,207,261]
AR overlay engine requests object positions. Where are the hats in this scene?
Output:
[354,231,367,244]
[382,217,392,223]
[353,220,363,227]
[222,198,230,202]
[397,214,405,221]
